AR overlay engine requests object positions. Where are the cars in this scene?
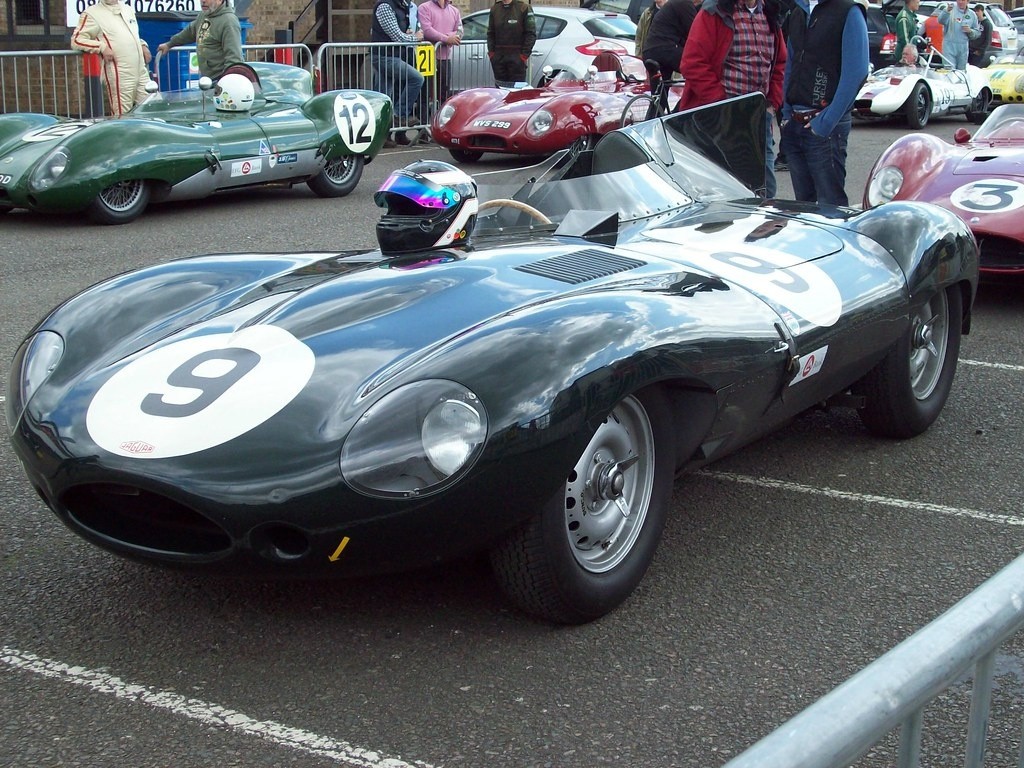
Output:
[806,1,1024,70]
[979,44,1024,108]
[448,8,639,98]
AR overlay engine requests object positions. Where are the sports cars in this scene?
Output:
[861,104,1024,281]
[429,74,689,164]
[849,37,993,131]
[1,59,396,227]
[4,116,980,629]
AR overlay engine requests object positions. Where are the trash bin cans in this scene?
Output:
[136,13,254,92]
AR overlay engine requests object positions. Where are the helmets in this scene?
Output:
[374,158,479,254]
[379,245,473,271]
[213,74,254,111]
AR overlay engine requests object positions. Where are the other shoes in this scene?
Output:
[382,138,396,148]
[395,135,411,145]
[394,117,420,127]
[774,158,790,172]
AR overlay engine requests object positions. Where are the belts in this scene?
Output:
[791,109,820,124]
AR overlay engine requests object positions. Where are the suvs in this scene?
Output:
[883,1,1019,59]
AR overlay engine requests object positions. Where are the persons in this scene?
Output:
[781,0,869,207]
[70,0,152,116]
[487,0,537,88]
[369,0,464,148]
[157,0,243,81]
[635,0,704,120]
[679,0,787,198]
[894,0,993,69]
